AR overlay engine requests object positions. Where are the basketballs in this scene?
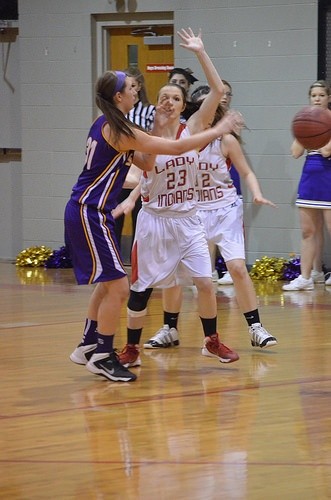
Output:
[291,107,331,150]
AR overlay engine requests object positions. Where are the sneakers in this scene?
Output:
[143,324,180,349]
[202,333,239,364]
[248,323,279,349]
[115,343,142,368]
[85,348,137,382]
[212,270,219,281]
[311,270,325,283]
[69,342,100,366]
[217,271,234,285]
[282,275,314,290]
[325,273,331,285]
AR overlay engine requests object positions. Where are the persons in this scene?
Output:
[67,68,246,385]
[109,97,277,351]
[113,65,156,262]
[310,205,324,283]
[280,81,331,291]
[188,85,212,102]
[115,26,240,370]
[164,64,198,97]
[207,78,246,286]
